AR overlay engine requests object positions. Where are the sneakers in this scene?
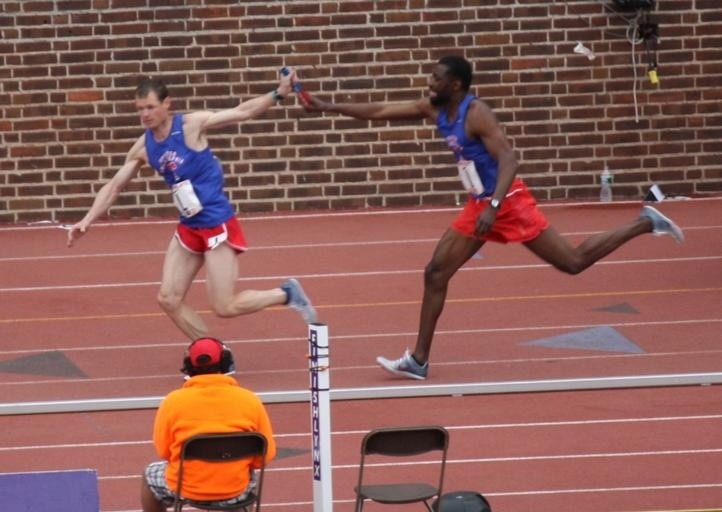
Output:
[639,204,684,245]
[280,278,316,322]
[376,349,428,380]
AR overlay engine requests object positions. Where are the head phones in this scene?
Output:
[180,337,234,375]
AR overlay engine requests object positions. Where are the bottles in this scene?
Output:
[600,166,613,202]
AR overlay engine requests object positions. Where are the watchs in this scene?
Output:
[490,198,503,209]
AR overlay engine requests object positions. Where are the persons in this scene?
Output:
[140,337,276,511]
[66,65,320,380]
[304,54,686,380]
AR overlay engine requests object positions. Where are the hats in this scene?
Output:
[187,336,223,368]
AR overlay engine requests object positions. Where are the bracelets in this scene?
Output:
[271,88,284,100]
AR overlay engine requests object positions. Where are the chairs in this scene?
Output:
[163,432,267,512]
[352,426,451,511]
